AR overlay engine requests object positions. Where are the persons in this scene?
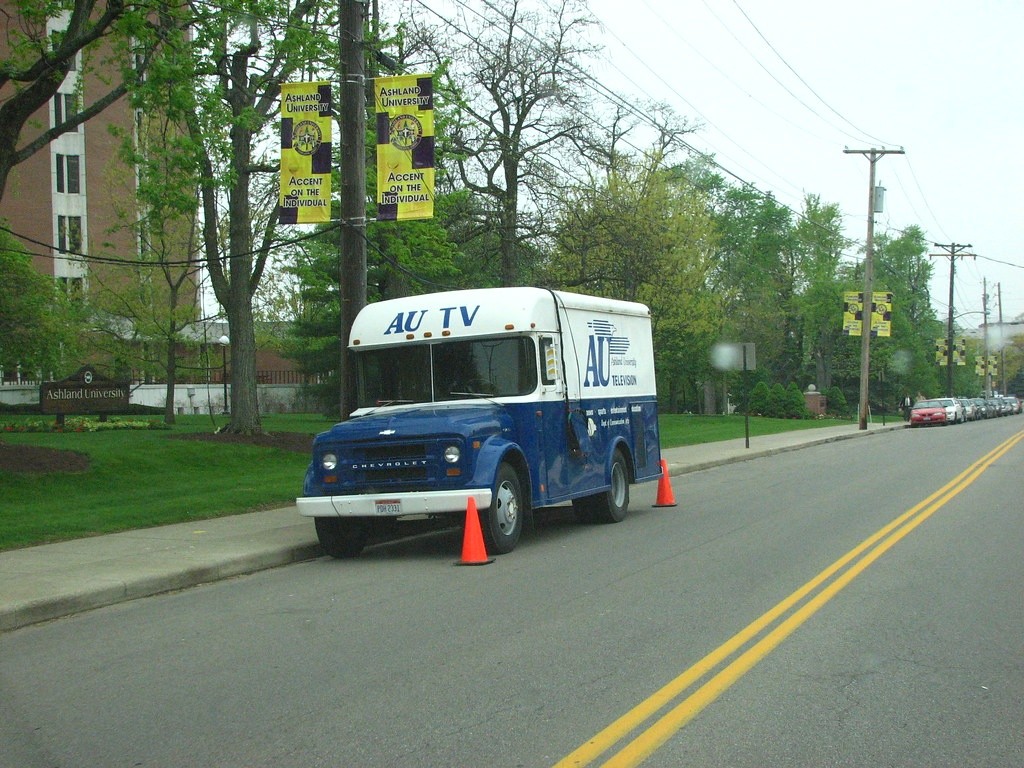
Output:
[900,393,913,422]
[915,392,925,402]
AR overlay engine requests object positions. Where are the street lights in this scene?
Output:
[219,334,231,414]
[946,311,990,397]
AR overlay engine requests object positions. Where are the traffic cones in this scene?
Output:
[453,495,496,565]
[653,458,678,508]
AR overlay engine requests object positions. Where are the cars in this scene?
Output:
[908,399,948,427]
[931,397,966,424]
[955,397,1023,421]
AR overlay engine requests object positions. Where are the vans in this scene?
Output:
[293,284,664,561]
